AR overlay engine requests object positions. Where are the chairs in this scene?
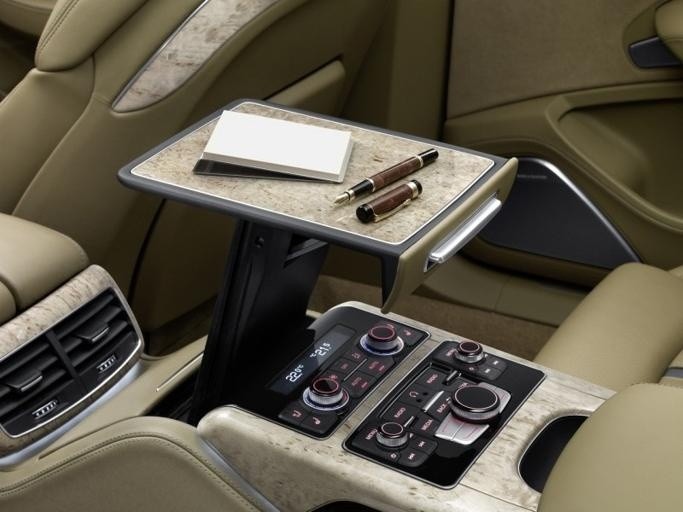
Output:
[532,259,683,391]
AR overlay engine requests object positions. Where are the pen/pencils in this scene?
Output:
[334,148,439,206]
